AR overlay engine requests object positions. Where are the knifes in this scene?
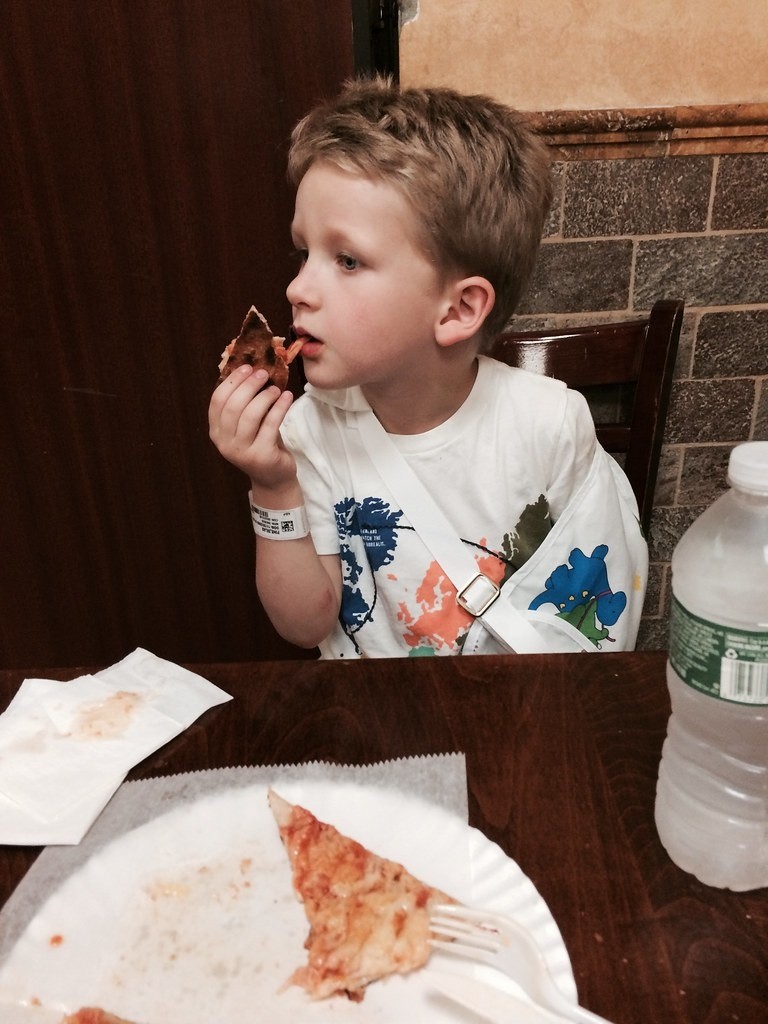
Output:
[422,970,557,1024]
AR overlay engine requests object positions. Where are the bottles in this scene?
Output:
[655,442,768,891]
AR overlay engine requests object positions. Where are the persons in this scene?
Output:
[207,75,649,663]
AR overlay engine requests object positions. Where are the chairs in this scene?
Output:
[481,299,686,538]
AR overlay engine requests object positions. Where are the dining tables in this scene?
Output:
[0,650,768,1024]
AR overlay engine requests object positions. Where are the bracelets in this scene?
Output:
[248,490,311,541]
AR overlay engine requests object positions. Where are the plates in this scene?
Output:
[1,786,577,1024]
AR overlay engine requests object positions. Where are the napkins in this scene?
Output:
[1,646,234,846]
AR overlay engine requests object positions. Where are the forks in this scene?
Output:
[426,905,615,1024]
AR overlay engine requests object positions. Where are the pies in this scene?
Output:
[218,304,313,391]
[266,791,481,999]
